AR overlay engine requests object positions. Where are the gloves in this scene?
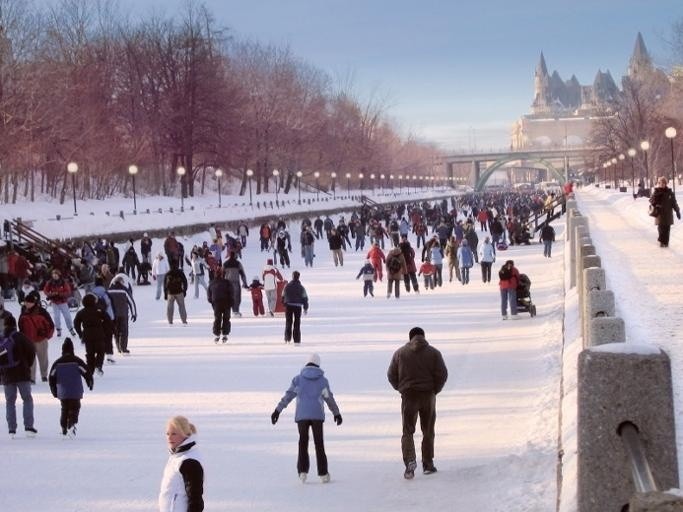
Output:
[270,409,280,425]
[334,414,343,426]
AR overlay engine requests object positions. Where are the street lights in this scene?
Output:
[664,126,676,199]
[67,161,84,217]
[246,167,255,206]
[272,167,469,208]
[174,165,186,209]
[214,167,224,205]
[129,162,140,211]
[640,141,651,196]
[603,148,636,194]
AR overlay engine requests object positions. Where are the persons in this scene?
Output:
[539,220,555,258]
[457,187,566,246]
[649,176,680,247]
[271,353,345,483]
[207,267,234,343]
[247,274,265,317]
[260,217,292,269]
[387,326,448,477]
[497,259,520,318]
[122,232,152,286]
[281,271,308,345]
[1,291,54,435]
[44,269,76,337]
[48,337,94,436]
[1,238,120,300]
[155,414,204,510]
[300,200,495,299]
[152,220,249,324]
[262,258,283,317]
[73,267,136,376]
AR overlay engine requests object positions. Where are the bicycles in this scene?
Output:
[37,294,81,313]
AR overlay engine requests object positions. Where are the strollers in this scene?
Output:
[513,274,539,317]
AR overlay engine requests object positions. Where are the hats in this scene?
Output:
[3,315,16,328]
[408,327,426,341]
[61,337,74,353]
[304,351,322,368]
[51,268,61,276]
[24,295,36,303]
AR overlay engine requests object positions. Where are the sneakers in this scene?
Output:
[403,459,417,479]
[511,314,522,320]
[24,426,38,434]
[8,430,17,435]
[320,473,331,484]
[298,472,309,482]
[502,315,509,321]
[422,465,438,474]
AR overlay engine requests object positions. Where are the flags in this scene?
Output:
[274,280,288,312]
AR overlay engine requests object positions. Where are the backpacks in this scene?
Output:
[499,264,513,281]
